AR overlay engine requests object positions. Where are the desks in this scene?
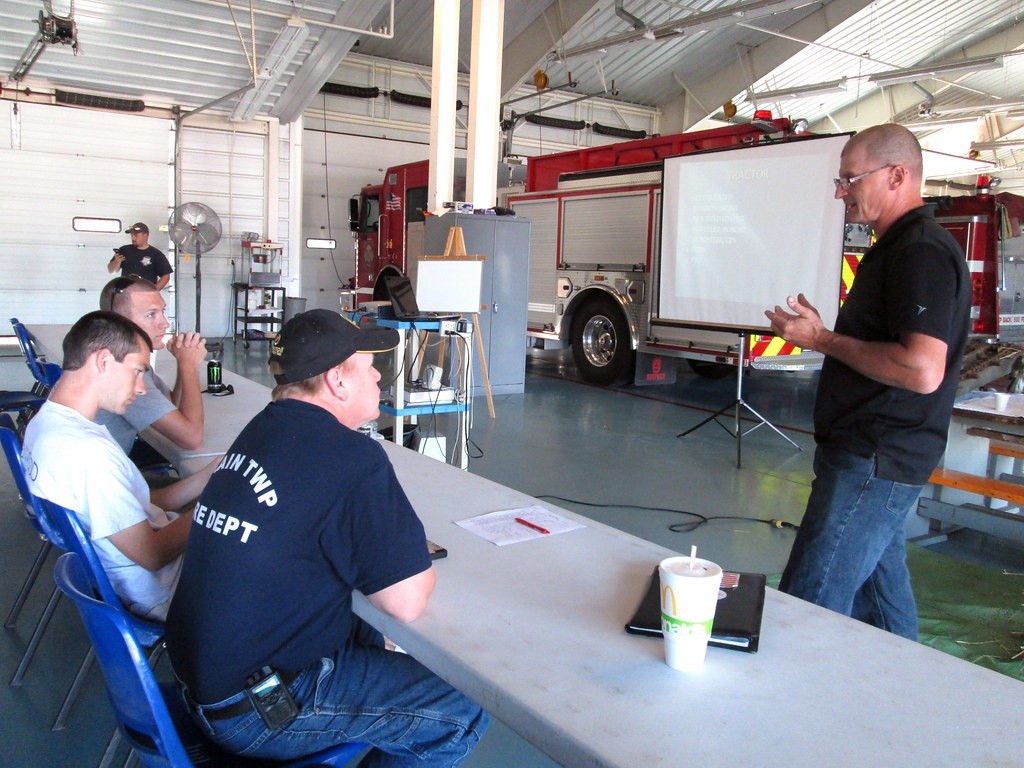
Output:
[141,345,1024,768]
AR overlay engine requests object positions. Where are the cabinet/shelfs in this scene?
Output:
[231,284,287,348]
[378,318,474,470]
[425,216,532,395]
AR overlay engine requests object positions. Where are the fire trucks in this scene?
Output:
[349,111,877,391]
[921,175,1024,344]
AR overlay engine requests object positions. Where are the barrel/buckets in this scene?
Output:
[284,296,307,324]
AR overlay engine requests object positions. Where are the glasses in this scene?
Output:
[833,163,907,191]
[109,274,142,312]
[201,384,234,397]
[129,226,142,231]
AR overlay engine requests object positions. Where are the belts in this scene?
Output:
[171,670,300,723]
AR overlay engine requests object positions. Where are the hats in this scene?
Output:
[268,309,400,385]
[125,222,149,233]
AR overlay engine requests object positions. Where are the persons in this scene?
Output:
[24,224,228,622]
[161,309,490,768]
[765,123,972,641]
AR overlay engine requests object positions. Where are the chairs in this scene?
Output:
[0,318,372,768]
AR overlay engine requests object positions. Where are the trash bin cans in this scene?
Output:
[284,297,307,324]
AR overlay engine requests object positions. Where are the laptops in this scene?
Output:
[384,274,461,323]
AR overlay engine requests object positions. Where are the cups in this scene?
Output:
[658,557,723,671]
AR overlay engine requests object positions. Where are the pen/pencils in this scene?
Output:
[514,517,550,534]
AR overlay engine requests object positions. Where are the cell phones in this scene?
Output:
[113,248,123,256]
[426,538,448,560]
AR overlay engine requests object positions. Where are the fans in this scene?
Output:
[168,200,221,334]
